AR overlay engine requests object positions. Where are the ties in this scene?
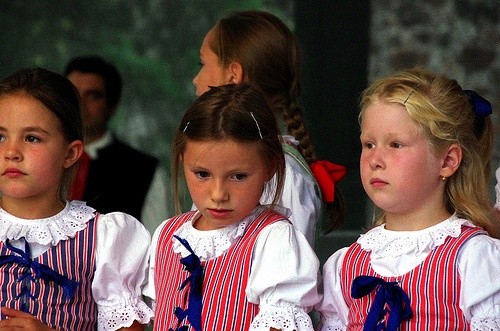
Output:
[68,150,89,200]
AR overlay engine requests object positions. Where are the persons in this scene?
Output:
[59,50,158,222]
[317,69,500,330]
[153,80,322,331]
[0,67,152,331]
[191,12,347,257]
